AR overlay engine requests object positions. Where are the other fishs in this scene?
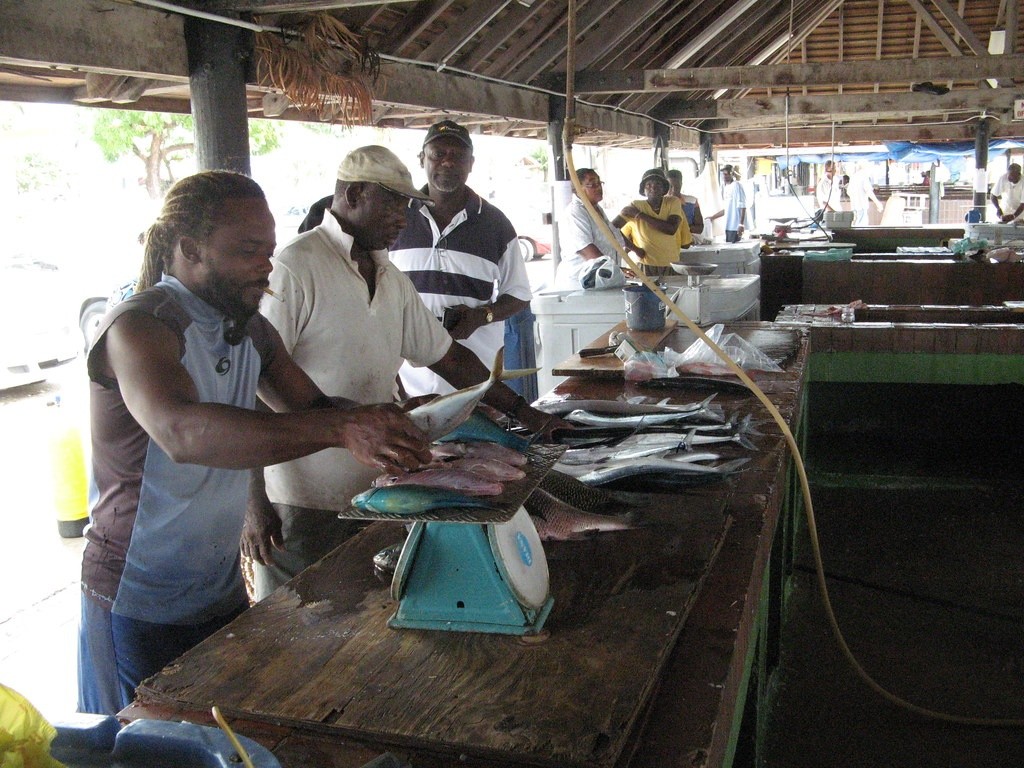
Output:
[348,358,766,589]
[581,259,612,290]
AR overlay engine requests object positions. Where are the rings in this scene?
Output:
[627,271,630,273]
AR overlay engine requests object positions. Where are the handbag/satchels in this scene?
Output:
[578,255,625,290]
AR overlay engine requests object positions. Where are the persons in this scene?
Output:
[990,163,1024,223]
[847,159,883,227]
[816,160,849,221]
[704,165,748,244]
[554,168,636,278]
[74,170,433,716]
[666,170,704,234]
[913,171,930,186]
[240,146,573,605]
[881,195,906,228]
[299,121,530,420]
[612,169,692,277]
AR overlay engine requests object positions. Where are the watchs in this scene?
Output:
[478,309,494,324]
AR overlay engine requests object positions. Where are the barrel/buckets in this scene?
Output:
[622,285,667,329]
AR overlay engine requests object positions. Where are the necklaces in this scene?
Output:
[651,206,660,211]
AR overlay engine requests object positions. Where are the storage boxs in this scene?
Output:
[824,209,854,230]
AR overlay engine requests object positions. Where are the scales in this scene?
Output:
[960,205,987,222]
[336,443,573,636]
[666,262,718,327]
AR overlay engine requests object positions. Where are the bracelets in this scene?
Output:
[737,224,745,228]
[1011,214,1015,220]
[505,395,526,419]
[634,212,643,220]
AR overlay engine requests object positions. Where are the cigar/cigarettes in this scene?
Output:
[262,287,284,302]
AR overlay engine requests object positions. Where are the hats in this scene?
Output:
[337,146,436,207]
[639,168,670,196]
[719,165,733,171]
[422,119,473,153]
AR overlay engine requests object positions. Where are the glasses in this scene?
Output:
[580,181,605,188]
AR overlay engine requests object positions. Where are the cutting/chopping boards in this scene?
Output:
[552,320,678,377]
